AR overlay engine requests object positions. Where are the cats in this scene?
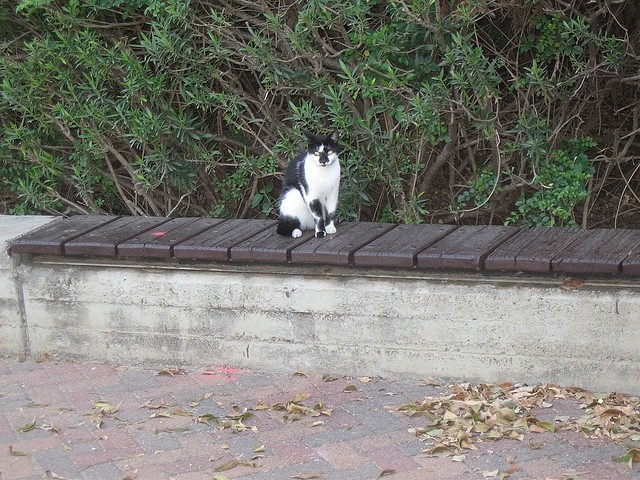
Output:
[275,128,342,239]
